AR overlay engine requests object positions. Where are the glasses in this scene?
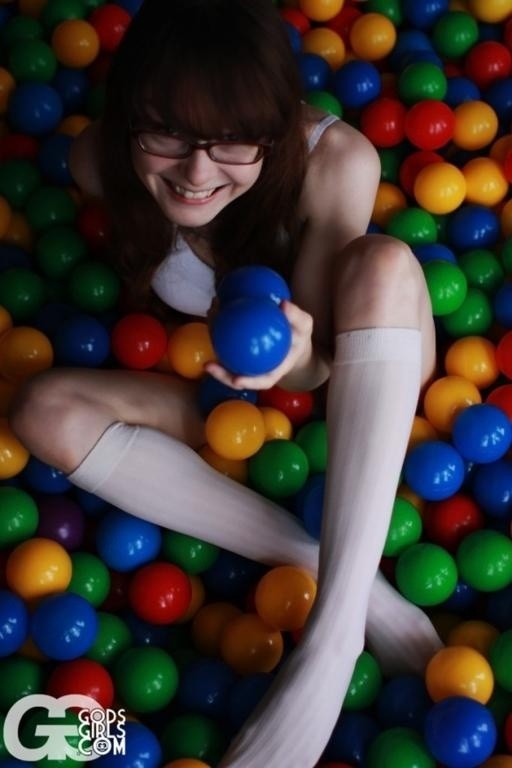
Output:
[135,126,269,167]
